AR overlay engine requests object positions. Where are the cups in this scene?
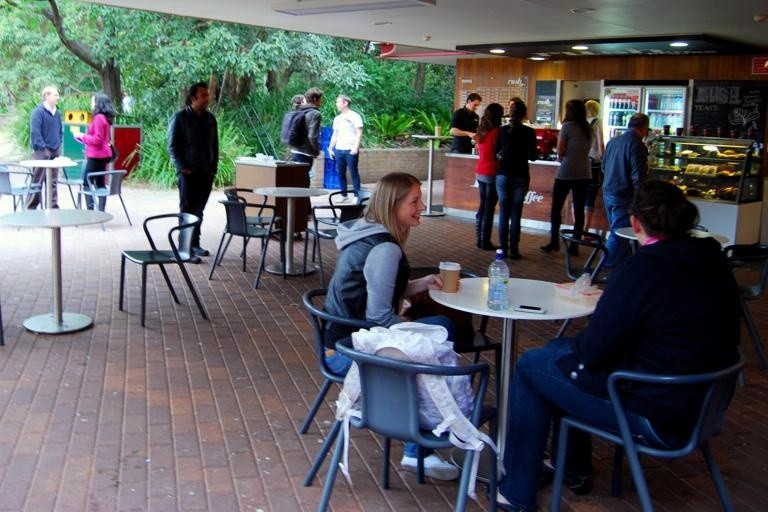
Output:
[437,261,461,293]
[653,125,756,139]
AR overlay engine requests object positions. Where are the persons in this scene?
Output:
[289,94,307,111]
[19,83,63,213]
[289,85,324,216]
[326,93,365,205]
[570,99,605,243]
[71,93,118,213]
[447,93,482,156]
[492,98,540,263]
[537,98,593,257]
[482,178,746,512]
[472,102,503,253]
[502,96,521,119]
[317,171,467,487]
[166,78,221,267]
[596,111,652,278]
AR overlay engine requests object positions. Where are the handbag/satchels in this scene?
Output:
[105,143,120,164]
[587,156,602,188]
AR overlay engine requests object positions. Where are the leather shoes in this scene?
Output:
[481,475,534,512]
[538,453,593,494]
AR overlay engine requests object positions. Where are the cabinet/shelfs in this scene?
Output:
[647,133,763,205]
[234,159,310,233]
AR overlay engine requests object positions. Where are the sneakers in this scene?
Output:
[190,246,209,256]
[335,195,349,203]
[189,255,201,263]
[399,451,460,481]
[476,241,579,260]
[352,197,359,204]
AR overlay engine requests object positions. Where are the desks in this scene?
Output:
[425,275,602,485]
[614,224,733,260]
[0,207,113,337]
[410,134,455,217]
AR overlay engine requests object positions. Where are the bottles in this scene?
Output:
[607,94,683,137]
[488,248,510,312]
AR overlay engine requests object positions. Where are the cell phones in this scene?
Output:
[514,304,546,314]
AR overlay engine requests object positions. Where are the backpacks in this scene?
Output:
[353,322,475,432]
[280,109,305,147]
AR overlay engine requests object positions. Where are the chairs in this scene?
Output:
[405,261,501,411]
[721,241,768,386]
[549,224,611,340]
[544,344,747,510]
[205,183,375,294]
[314,334,501,511]
[299,284,399,494]
[116,210,210,326]
[0,157,132,234]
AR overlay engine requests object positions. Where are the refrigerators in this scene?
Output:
[598,78,694,146]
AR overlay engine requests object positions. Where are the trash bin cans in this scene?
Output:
[319,127,343,190]
[62,110,94,179]
[103,124,143,185]
